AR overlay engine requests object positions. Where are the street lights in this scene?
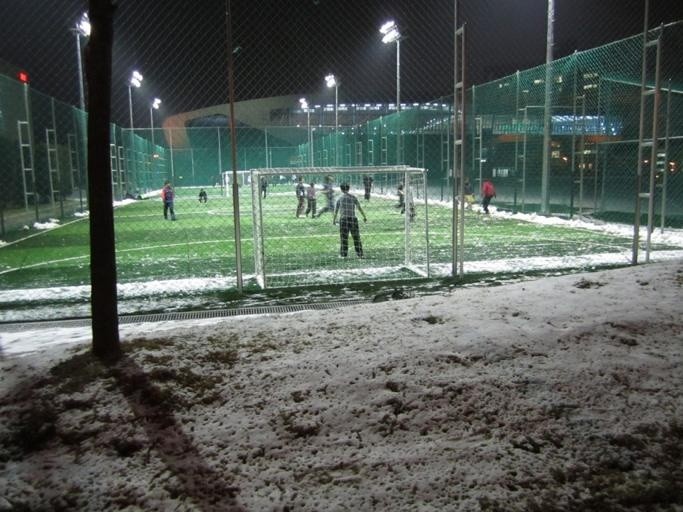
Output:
[150,97,163,160]
[73,10,93,190]
[377,19,404,190]
[298,97,311,166]
[128,69,144,195]
[324,72,340,166]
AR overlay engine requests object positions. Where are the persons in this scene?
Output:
[316,174,335,218]
[196,188,206,205]
[478,175,497,217]
[401,174,416,221]
[394,180,404,208]
[364,175,373,198]
[304,182,315,219]
[463,178,475,209]
[293,174,305,217]
[260,175,266,200]
[159,180,178,223]
[332,181,367,260]
